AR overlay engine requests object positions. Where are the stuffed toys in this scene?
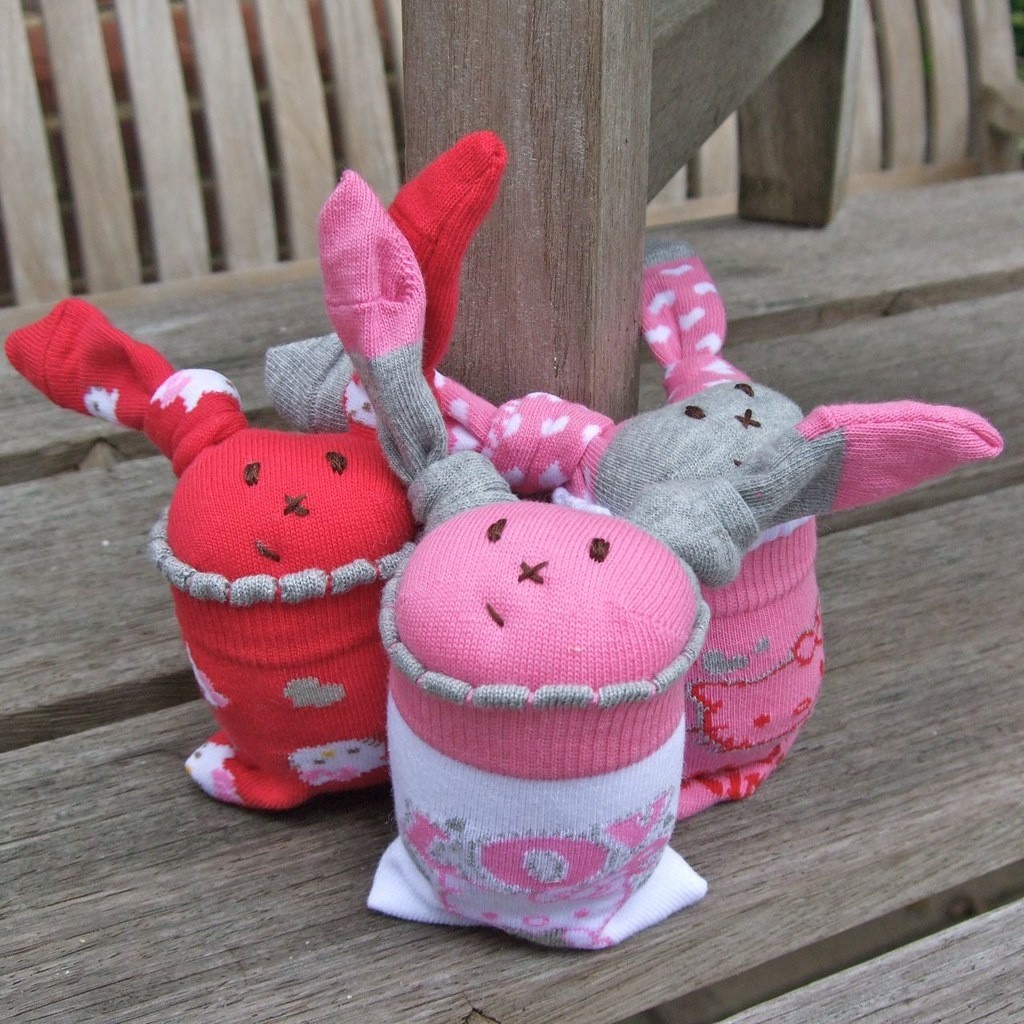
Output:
[263,239,1001,823]
[2,128,510,823]
[315,166,1003,954]
